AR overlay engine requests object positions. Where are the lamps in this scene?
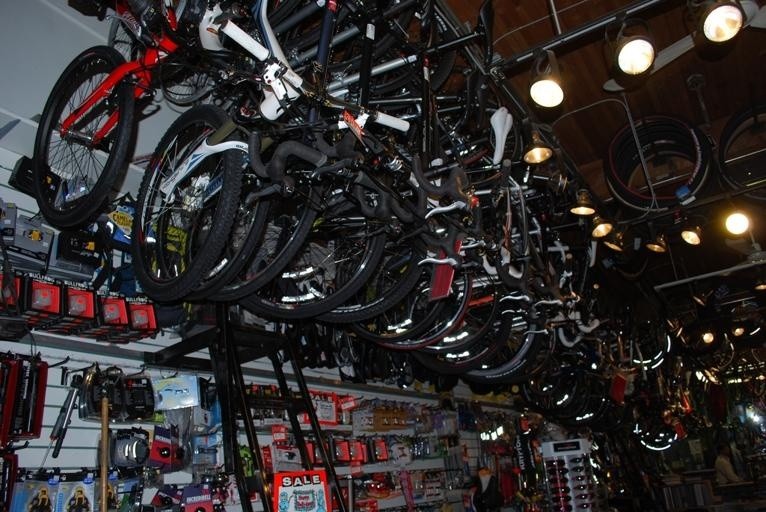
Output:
[613,20,655,76]
[725,209,751,238]
[592,214,612,237]
[524,128,552,163]
[647,230,666,252]
[604,230,625,252]
[666,277,766,344]
[529,50,565,107]
[681,222,702,244]
[726,363,765,385]
[570,187,596,215]
[696,3,746,43]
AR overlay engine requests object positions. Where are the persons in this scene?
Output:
[473,467,500,511]
[715,441,745,483]
